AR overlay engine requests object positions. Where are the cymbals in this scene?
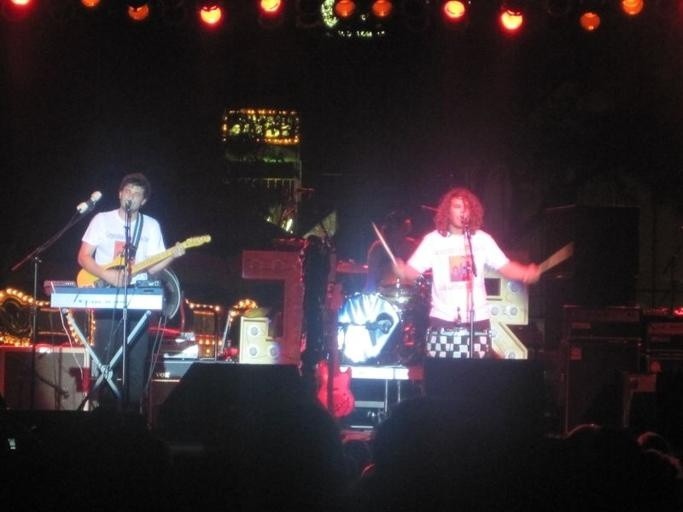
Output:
[336,263,374,274]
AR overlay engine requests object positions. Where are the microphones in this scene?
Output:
[76,190,102,214]
[460,214,468,234]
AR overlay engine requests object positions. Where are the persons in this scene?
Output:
[78,174,185,411]
[367,212,420,296]
[394,189,541,361]
[2,343,683,510]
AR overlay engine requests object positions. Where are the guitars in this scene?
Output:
[75,235,212,287]
[315,282,354,418]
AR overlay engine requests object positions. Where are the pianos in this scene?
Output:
[50,286,167,310]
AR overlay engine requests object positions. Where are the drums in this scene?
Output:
[381,284,416,313]
[335,291,405,364]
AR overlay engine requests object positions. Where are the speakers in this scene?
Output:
[545,204,639,308]
